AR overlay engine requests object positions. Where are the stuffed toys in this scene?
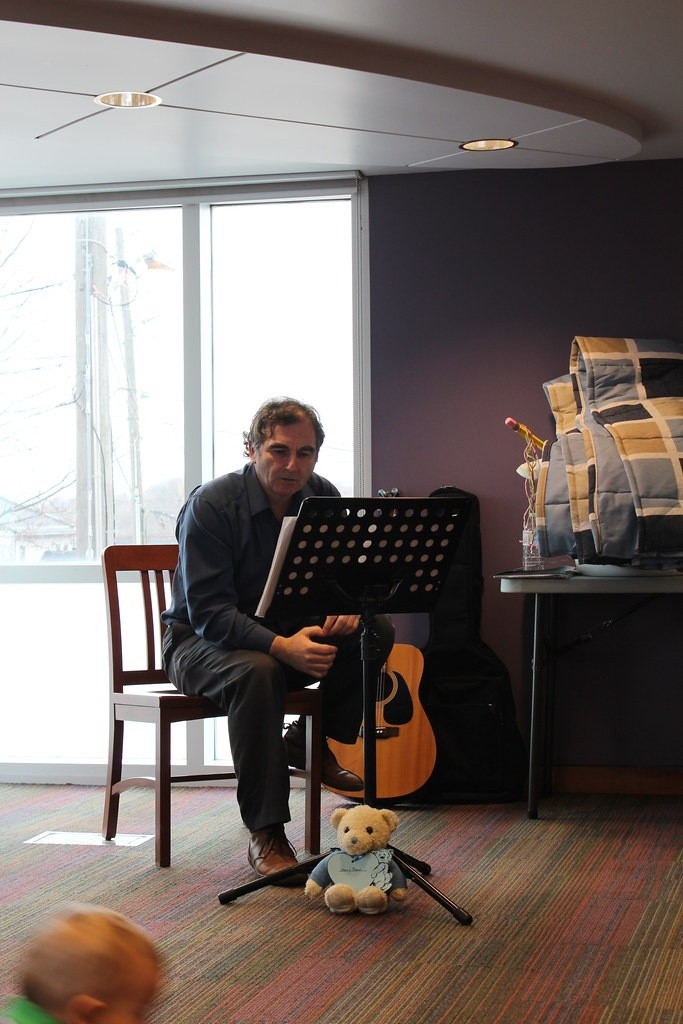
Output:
[304,805,407,914]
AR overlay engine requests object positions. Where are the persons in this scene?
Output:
[0,903,162,1024]
[163,397,397,884]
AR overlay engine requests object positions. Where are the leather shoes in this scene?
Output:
[247,824,307,886]
[282,720,363,792]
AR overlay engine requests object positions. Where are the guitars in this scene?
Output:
[318,487,439,804]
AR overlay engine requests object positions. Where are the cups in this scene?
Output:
[522,530,543,567]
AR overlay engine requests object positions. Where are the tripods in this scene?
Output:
[215,496,476,929]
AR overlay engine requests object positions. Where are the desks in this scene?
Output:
[499,574,683,820]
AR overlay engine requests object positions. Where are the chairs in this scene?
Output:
[101,544,324,869]
